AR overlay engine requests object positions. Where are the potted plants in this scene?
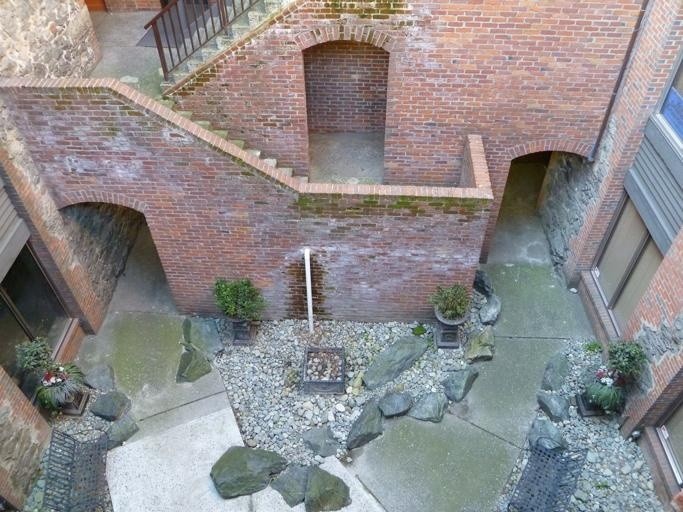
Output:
[212,277,267,347]
[426,280,472,352]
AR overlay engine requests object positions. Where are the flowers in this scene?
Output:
[585,340,645,414]
[13,337,86,413]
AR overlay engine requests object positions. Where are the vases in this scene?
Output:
[574,388,604,418]
[58,387,88,416]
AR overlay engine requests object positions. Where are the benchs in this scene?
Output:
[507,437,588,512]
[41,426,123,512]
